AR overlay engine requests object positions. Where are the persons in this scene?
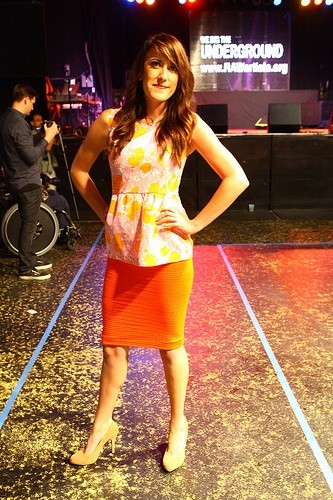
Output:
[0,83,58,280]
[70,33,250,474]
[27,113,61,191]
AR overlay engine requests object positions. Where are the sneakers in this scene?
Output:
[30,257,53,270]
[18,267,51,281]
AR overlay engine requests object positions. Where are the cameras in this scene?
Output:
[36,120,53,133]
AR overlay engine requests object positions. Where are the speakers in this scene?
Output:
[268,103,301,133]
[197,103,228,134]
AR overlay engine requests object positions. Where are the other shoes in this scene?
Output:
[59,235,76,246]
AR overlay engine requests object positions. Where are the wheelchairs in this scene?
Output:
[2,171,83,258]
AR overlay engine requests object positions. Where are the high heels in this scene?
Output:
[70,418,120,466]
[161,420,189,473]
